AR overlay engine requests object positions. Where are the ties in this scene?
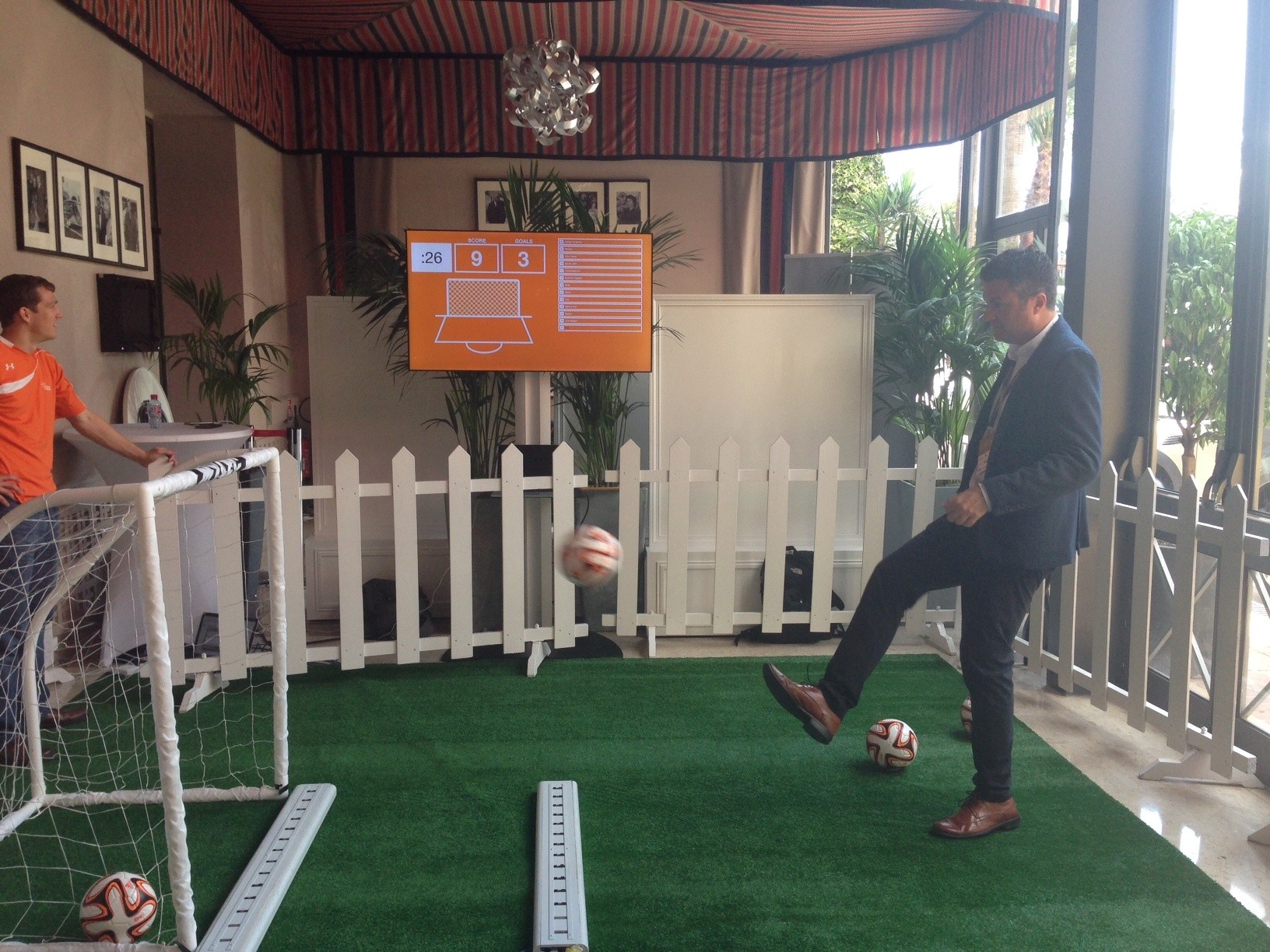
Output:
[976,362,1026,487]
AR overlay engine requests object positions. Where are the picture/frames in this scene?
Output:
[12,136,59,256]
[607,179,651,233]
[87,163,122,267]
[563,179,608,235]
[474,178,525,232]
[53,152,93,264]
[114,173,149,272]
[523,180,563,233]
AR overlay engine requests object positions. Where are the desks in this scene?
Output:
[63,419,253,669]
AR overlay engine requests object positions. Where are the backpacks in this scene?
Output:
[756,547,846,644]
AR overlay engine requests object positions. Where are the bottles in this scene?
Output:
[148,395,162,429]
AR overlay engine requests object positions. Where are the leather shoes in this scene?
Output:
[760,658,842,744]
[44,709,87,726]
[0,740,54,767]
[933,793,1021,840]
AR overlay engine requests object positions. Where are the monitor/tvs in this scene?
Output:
[96,273,162,354]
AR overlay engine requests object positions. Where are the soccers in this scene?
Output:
[961,695,972,735]
[561,524,623,589]
[866,718,918,770]
[79,871,158,943]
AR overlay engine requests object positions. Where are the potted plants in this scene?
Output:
[824,173,1012,624]
[309,161,705,625]
[158,273,294,601]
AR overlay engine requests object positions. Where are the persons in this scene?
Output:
[762,246,1102,840]
[486,195,641,223]
[0,274,179,763]
